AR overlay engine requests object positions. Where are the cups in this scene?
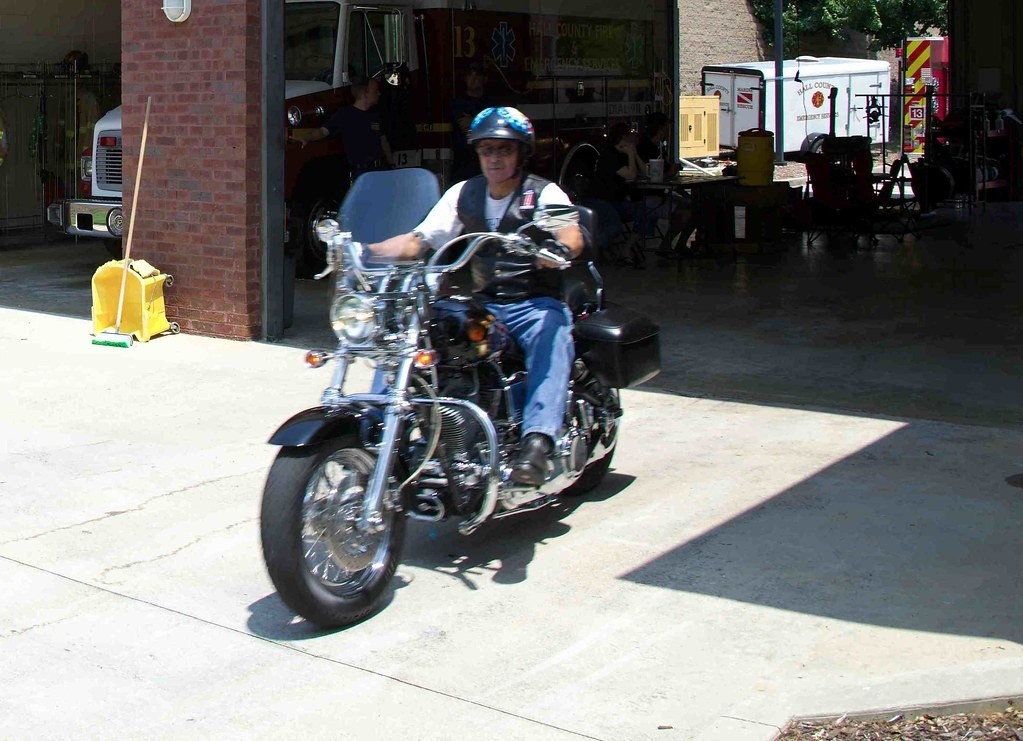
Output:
[645,159,664,184]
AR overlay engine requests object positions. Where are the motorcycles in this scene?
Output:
[256,165,667,632]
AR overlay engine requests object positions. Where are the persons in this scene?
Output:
[329,106,586,487]
[443,61,492,188]
[288,76,396,190]
[636,111,697,262]
[594,122,659,266]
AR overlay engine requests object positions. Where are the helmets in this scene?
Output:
[467,107,536,145]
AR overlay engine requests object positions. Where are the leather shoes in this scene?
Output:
[511,433,548,486]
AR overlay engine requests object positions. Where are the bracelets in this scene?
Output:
[388,164,396,168]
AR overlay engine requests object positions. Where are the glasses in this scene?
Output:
[477,143,517,157]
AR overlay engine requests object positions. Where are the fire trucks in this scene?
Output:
[46,1,680,270]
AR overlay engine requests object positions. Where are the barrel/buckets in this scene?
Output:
[737,128,773,186]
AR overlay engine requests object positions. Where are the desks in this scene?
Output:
[624,175,747,273]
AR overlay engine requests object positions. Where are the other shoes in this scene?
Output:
[673,243,689,252]
[656,247,670,260]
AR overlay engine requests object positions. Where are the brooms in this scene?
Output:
[91,95,152,348]
